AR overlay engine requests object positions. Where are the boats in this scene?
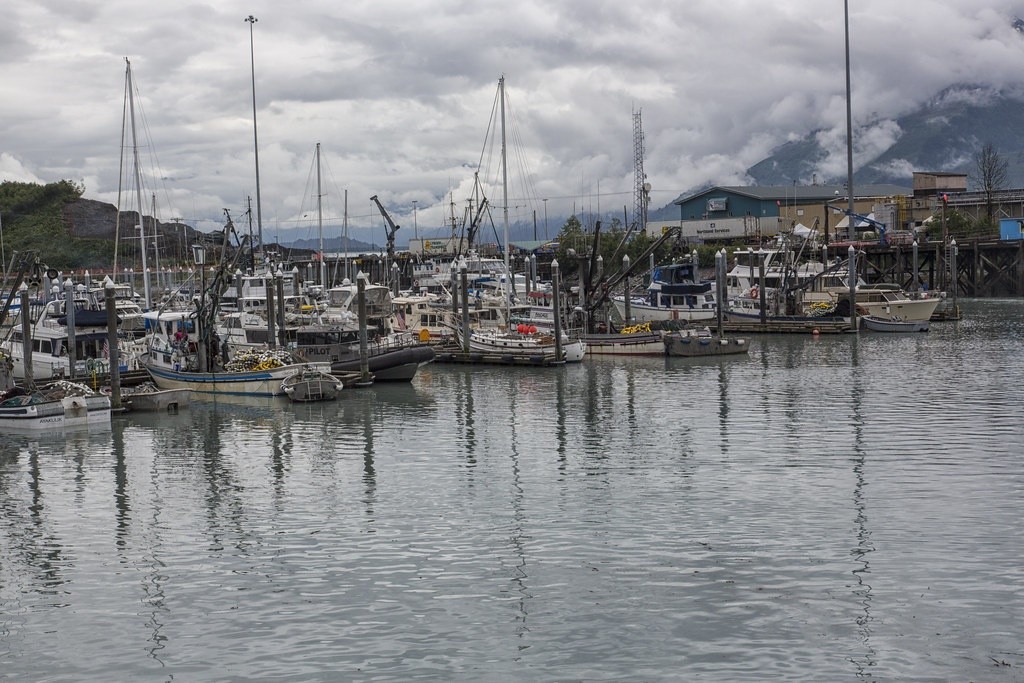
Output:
[1,381,111,429]
[275,317,438,382]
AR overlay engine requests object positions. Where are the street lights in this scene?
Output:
[241,13,265,256]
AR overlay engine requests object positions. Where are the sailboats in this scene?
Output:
[0,57,944,432]
[436,74,587,365]
[137,266,345,404]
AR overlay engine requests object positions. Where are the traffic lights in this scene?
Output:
[939,193,949,203]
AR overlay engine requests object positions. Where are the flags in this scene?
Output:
[395,311,405,330]
[103,342,108,359]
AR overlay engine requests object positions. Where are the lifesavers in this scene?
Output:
[750,288,759,298]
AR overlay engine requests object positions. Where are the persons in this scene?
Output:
[517,322,537,334]
[174,332,188,343]
